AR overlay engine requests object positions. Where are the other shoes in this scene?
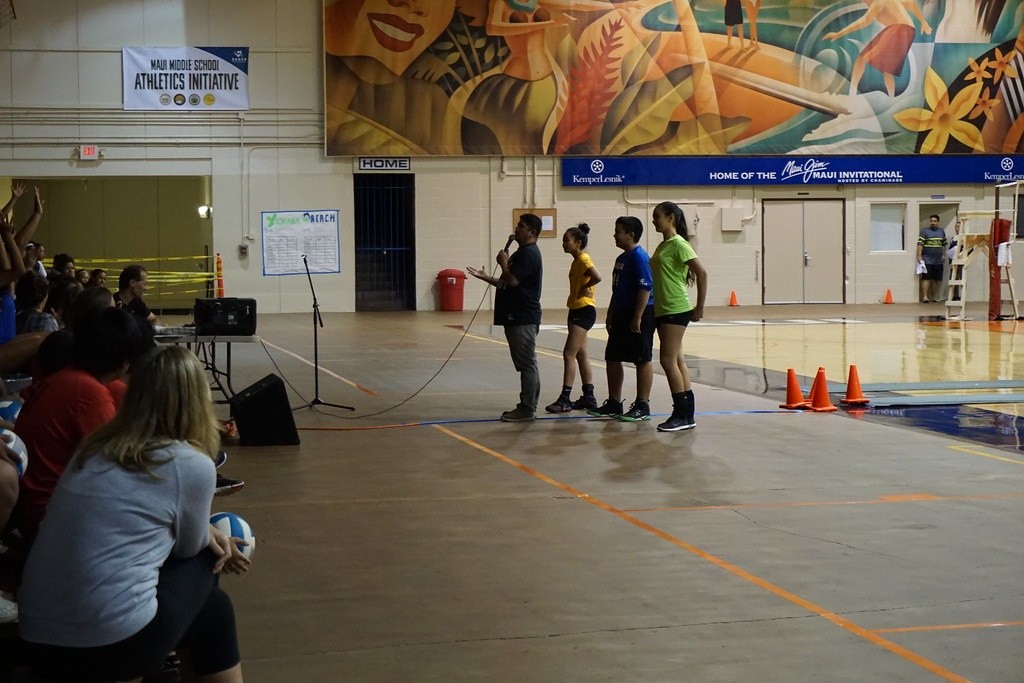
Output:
[213,450,228,468]
[931,297,940,302]
[140,655,185,683]
[0,596,19,624]
[922,298,930,303]
[502,405,537,421]
[214,473,245,497]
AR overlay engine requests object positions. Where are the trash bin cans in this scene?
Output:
[435,269,468,311]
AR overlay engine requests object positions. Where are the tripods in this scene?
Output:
[291,255,355,411]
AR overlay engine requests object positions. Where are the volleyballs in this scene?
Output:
[211,511,257,560]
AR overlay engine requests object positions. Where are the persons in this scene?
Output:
[0,180,251,683]
[947,222,962,301]
[648,202,708,432]
[586,216,656,421]
[466,213,543,422]
[546,222,599,413]
[917,215,948,303]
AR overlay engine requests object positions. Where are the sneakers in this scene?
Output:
[656,414,696,431]
[587,396,623,417]
[617,397,652,420]
[572,396,598,410]
[545,394,573,413]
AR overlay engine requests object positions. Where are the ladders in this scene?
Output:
[945,231,1020,320]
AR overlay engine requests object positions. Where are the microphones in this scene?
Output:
[504,234,515,252]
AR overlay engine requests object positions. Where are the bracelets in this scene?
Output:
[587,283,591,291]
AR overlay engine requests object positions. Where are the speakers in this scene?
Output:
[229,374,301,446]
